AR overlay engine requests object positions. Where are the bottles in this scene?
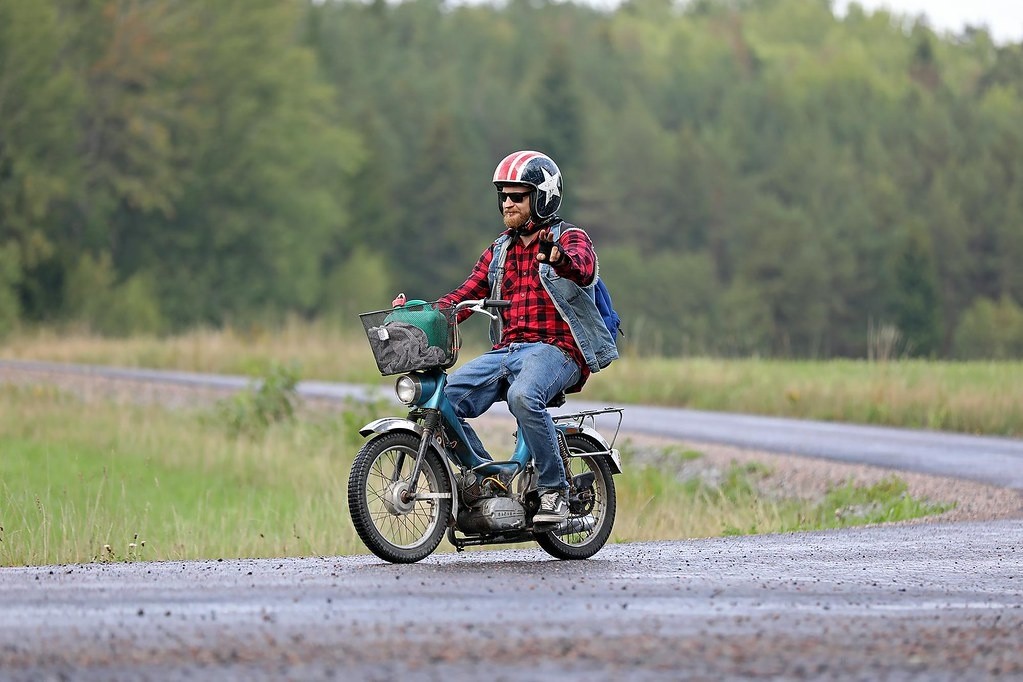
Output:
[383,292,447,356]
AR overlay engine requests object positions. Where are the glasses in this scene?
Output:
[497,190,533,203]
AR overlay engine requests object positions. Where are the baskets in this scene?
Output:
[359,301,457,377]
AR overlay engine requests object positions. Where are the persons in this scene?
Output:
[431,150,619,523]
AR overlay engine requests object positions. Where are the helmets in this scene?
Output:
[492,149,563,218]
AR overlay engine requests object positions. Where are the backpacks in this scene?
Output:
[560,222,621,341]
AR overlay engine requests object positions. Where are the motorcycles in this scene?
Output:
[346,297,625,565]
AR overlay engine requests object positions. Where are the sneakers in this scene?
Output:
[532,490,571,524]
